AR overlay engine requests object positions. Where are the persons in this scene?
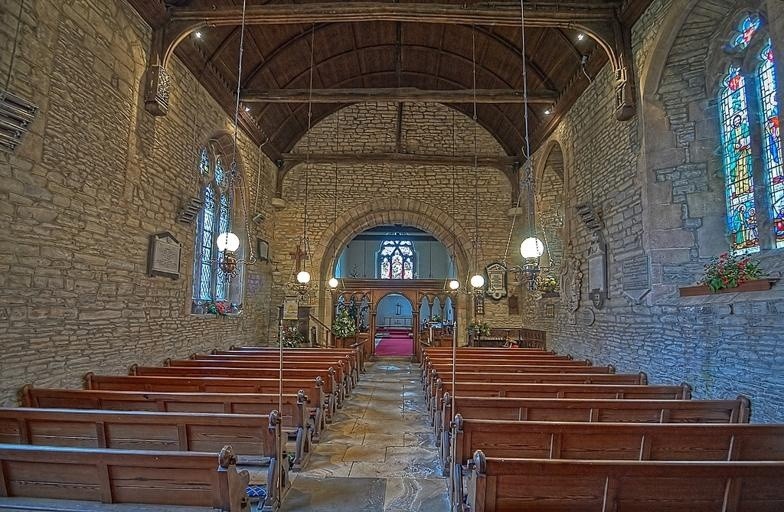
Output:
[731,203,749,244]
[727,115,752,199]
[774,208,784,239]
[747,207,760,241]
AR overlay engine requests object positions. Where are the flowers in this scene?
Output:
[543,271,557,286]
[206,296,239,315]
[697,252,762,292]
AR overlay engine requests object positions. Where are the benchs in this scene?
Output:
[421,348,784,512]
[0,340,361,512]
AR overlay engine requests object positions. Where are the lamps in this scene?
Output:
[322,103,346,298]
[503,0,553,289]
[442,103,464,298]
[284,22,320,302]
[209,0,257,283]
[462,24,497,295]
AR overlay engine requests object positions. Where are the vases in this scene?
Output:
[543,286,554,292]
